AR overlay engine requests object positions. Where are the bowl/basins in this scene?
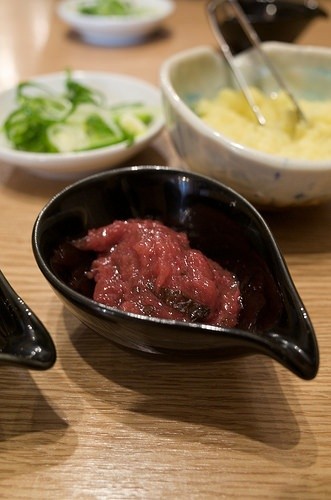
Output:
[159,41,331,208]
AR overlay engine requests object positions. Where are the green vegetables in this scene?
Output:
[3,75,154,155]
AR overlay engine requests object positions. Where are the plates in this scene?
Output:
[0,69,167,181]
[0,268,56,372]
[55,0,174,46]
[31,165,320,379]
